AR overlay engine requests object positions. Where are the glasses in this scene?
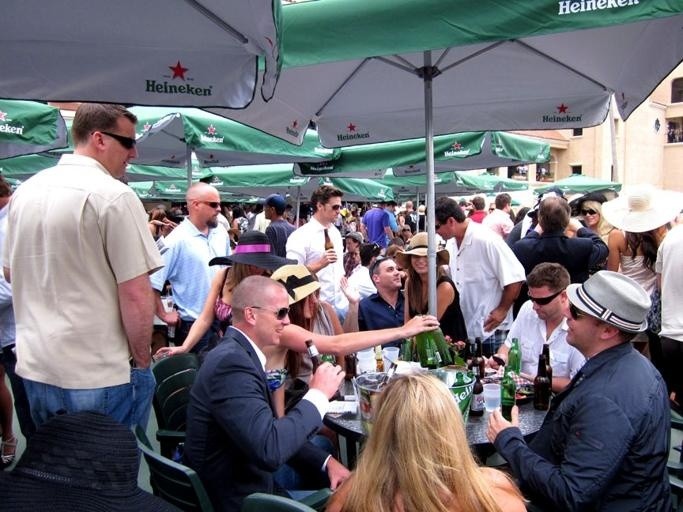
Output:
[325,203,341,210]
[581,209,597,216]
[525,286,566,305]
[196,199,221,209]
[250,304,291,321]
[435,221,442,229]
[90,128,137,150]
[372,254,390,274]
[567,302,595,321]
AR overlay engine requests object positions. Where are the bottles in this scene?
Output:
[323,229,336,262]
[532,354,550,411]
[304,313,521,422]
[541,344,553,395]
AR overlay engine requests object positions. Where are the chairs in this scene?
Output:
[133,352,336,512]
[667,399,682,512]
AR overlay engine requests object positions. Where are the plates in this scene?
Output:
[514,393,533,403]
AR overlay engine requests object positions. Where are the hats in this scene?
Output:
[526,185,566,219]
[566,187,620,219]
[563,268,654,334]
[0,409,185,512]
[256,193,287,208]
[207,229,299,270]
[269,263,322,307]
[599,183,683,234]
[395,231,450,270]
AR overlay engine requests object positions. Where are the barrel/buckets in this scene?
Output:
[350,372,399,434]
[428,367,477,435]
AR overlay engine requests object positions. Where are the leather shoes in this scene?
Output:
[0,433,19,469]
